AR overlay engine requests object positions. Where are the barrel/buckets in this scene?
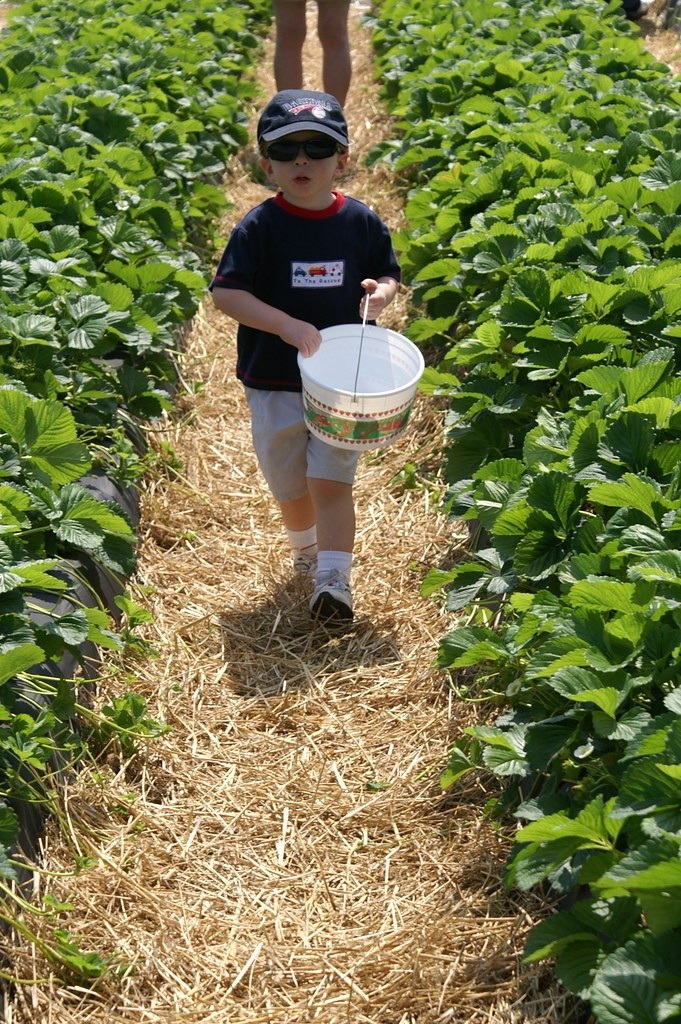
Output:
[297,292,425,452]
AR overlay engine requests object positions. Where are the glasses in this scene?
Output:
[267,134,343,163]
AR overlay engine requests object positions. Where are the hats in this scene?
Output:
[256,89,349,146]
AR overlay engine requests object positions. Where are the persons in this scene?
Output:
[210,90,402,630]
[272,0,352,109]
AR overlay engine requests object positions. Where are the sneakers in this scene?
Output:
[308,568,353,628]
[294,556,318,596]
